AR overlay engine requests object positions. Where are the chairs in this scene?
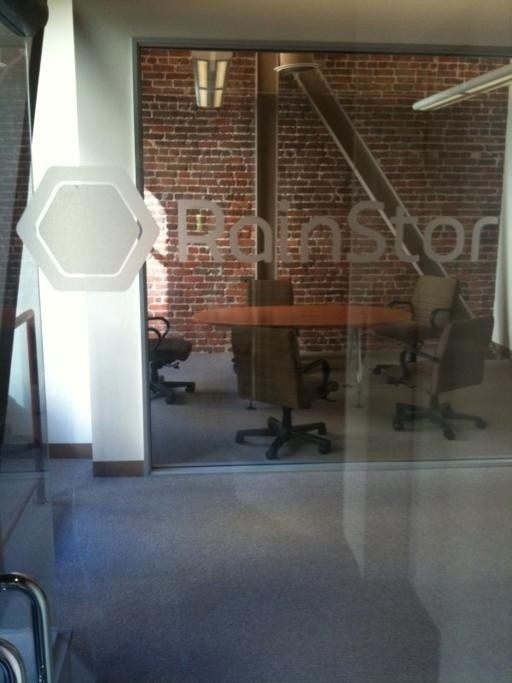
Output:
[384,313,494,440]
[147,315,195,404]
[373,274,458,386]
[229,325,332,460]
[247,279,339,408]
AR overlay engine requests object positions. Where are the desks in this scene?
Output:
[191,303,413,330]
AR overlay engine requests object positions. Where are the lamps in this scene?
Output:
[189,49,233,109]
[412,62,512,113]
